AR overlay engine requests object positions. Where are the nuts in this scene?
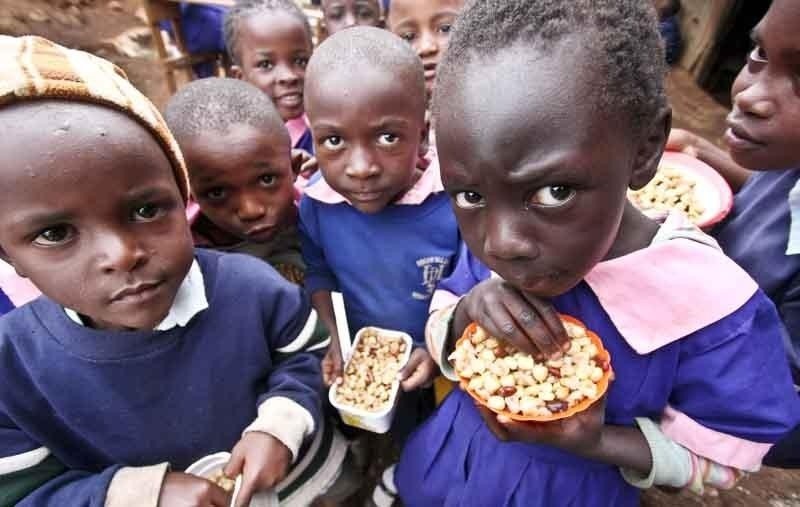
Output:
[335,329,408,411]
[450,313,606,418]
[208,467,237,491]
[627,165,706,223]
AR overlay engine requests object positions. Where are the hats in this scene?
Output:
[0,34,191,209]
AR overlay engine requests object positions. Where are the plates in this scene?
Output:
[628,151,734,230]
[454,311,612,423]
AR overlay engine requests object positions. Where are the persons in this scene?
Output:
[1,34,374,506]
[662,1,799,371]
[369,0,799,506]
[158,75,319,292]
[175,0,478,203]
[284,21,494,458]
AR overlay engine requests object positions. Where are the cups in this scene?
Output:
[185,451,280,507]
